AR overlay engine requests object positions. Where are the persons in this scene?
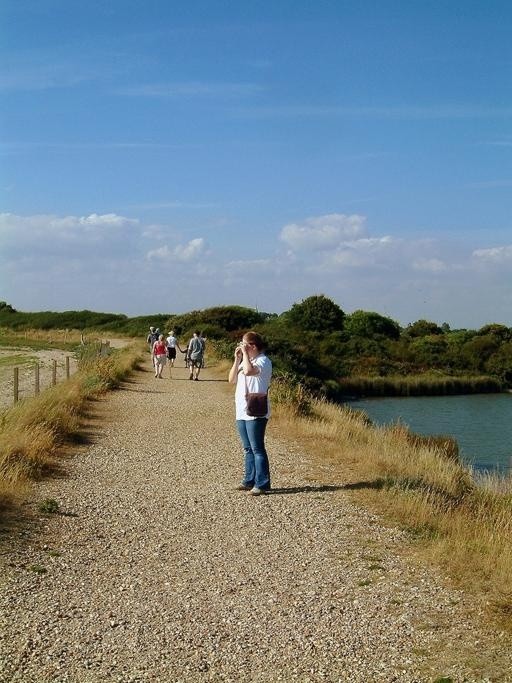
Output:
[147,327,205,380]
[229,331,272,495]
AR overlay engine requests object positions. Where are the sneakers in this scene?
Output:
[232,483,266,496]
[190,373,199,381]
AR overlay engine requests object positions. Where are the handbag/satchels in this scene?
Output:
[245,393,268,417]
